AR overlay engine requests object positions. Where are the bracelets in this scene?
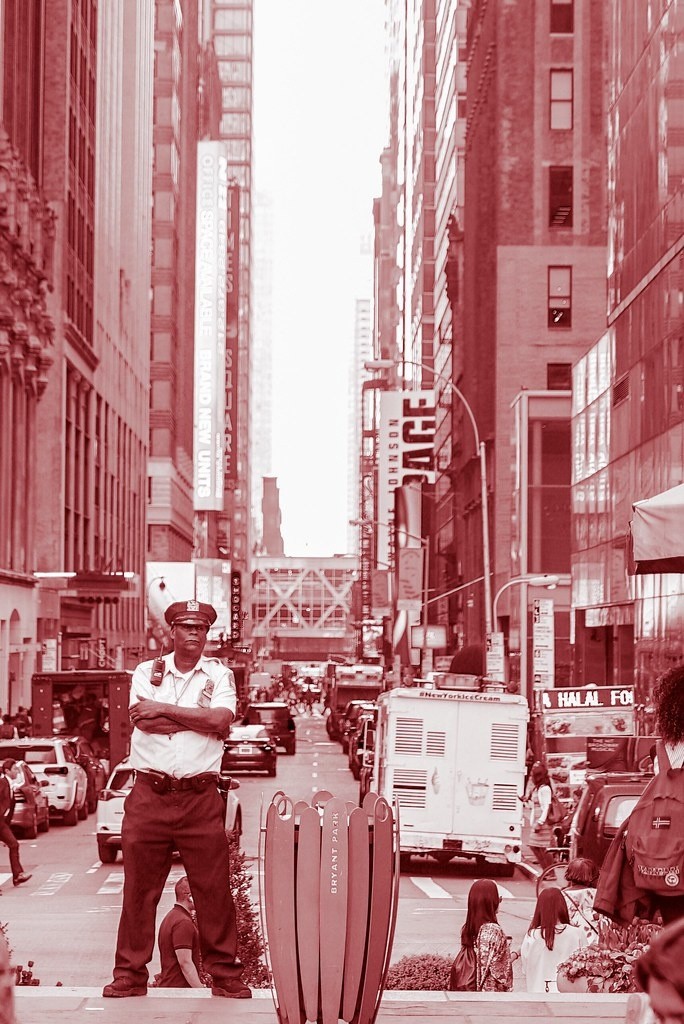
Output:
[512,951,519,959]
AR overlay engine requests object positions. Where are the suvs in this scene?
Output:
[94,756,243,865]
[0,735,91,827]
[56,737,108,815]
[238,703,306,756]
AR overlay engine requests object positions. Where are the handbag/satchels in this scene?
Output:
[544,784,567,826]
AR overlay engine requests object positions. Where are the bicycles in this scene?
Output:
[535,845,600,904]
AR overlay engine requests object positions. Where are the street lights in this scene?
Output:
[350,516,432,690]
[363,359,562,692]
[333,551,398,690]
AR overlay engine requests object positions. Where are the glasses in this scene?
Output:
[499,896,502,903]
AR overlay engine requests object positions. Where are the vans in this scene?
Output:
[568,771,656,883]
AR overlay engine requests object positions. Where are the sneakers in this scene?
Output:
[103,976,147,997]
[212,977,252,998]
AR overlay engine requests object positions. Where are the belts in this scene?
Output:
[136,772,217,790]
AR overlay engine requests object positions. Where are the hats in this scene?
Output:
[164,600,217,634]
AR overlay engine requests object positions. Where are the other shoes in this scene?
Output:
[538,875,556,880]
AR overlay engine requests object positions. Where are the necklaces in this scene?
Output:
[172,670,197,706]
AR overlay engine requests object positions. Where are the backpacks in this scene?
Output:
[625,741,684,897]
[451,941,477,991]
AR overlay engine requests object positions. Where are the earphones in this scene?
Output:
[190,897,193,901]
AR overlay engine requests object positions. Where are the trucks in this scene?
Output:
[30,667,133,789]
[359,669,531,877]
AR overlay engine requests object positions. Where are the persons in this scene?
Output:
[460,878,520,992]
[562,858,602,945]
[520,765,557,880]
[561,790,583,834]
[103,600,252,998]
[652,662,684,774]
[521,887,588,993]
[158,876,204,988]
[0,706,32,739]
[634,918,684,1024]
[256,685,330,716]
[0,758,33,887]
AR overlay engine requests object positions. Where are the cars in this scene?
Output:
[222,725,281,778]
[0,759,50,838]
[295,662,389,807]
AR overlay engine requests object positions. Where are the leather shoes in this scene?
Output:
[13,874,32,886]
